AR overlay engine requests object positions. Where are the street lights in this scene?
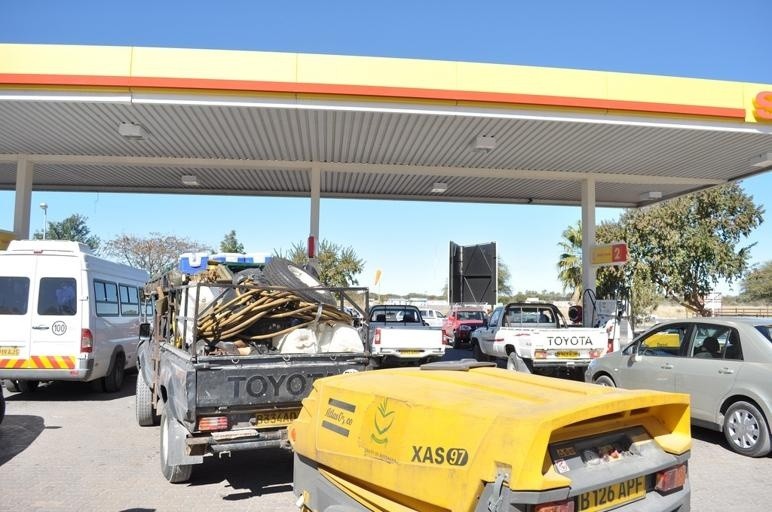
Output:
[40,203,48,240]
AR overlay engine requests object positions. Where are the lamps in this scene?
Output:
[118,123,148,142]
[640,192,662,201]
[751,152,772,168]
[430,183,448,194]
[181,175,202,186]
[471,136,495,156]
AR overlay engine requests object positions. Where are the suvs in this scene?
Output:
[441,310,488,349]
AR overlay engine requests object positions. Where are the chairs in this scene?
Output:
[376,314,386,321]
[695,331,739,359]
[401,313,413,322]
[539,315,549,323]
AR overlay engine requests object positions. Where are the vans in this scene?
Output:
[0,240,156,393]
[419,309,446,327]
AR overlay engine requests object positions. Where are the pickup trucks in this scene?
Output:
[136,282,369,482]
[369,303,443,370]
[470,303,609,368]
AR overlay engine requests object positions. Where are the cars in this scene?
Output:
[584,317,772,457]
